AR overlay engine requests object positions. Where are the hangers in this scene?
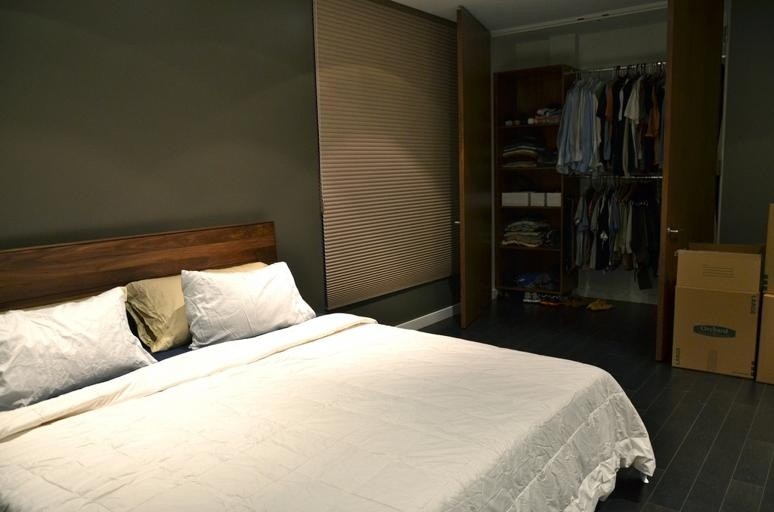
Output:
[570,60,668,92]
[579,171,663,207]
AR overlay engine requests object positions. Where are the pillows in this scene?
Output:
[181,260,316,350]
[123,261,270,354]
[0,284,157,410]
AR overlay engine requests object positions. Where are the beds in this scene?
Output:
[0,221,661,512]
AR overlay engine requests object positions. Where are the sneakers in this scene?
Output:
[523,292,613,310]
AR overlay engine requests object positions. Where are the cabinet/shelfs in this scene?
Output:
[456,0,725,363]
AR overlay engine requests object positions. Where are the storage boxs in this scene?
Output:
[672,241,763,380]
[755,293,774,384]
[762,203,774,295]
